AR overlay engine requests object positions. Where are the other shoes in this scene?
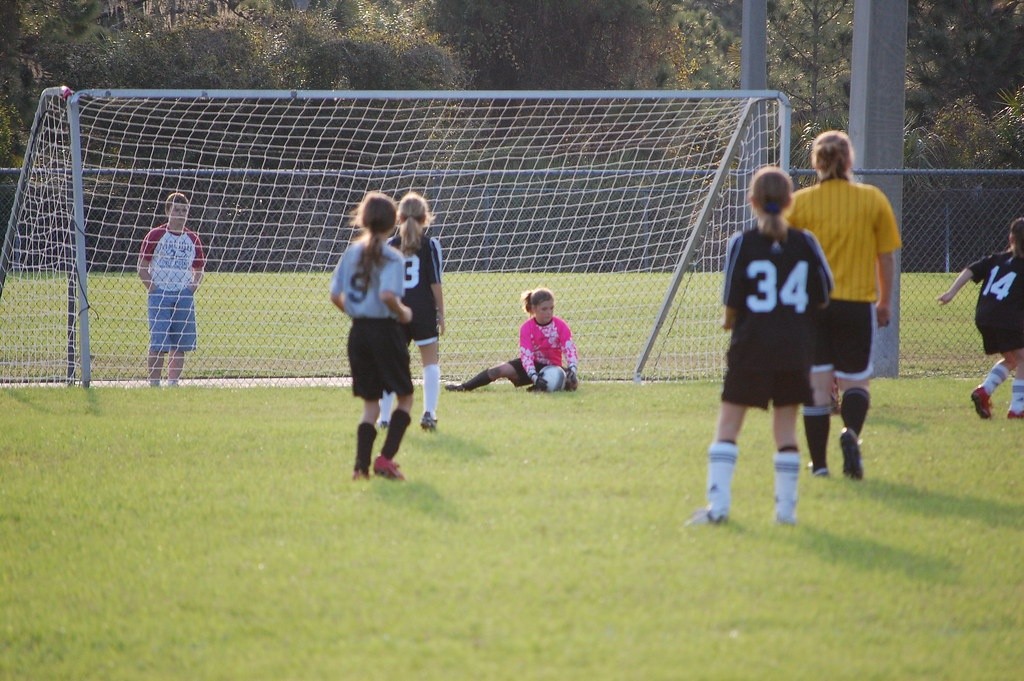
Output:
[377,421,390,428]
[444,382,466,391]
[684,505,727,526]
[420,412,437,430]
[840,428,865,479]
[812,469,830,478]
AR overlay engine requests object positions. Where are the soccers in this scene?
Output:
[538,364,568,392]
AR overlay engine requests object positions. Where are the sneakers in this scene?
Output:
[374,456,405,480]
[972,384,993,419]
[353,470,371,480]
[1008,410,1024,418]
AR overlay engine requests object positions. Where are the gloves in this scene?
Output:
[534,371,548,391]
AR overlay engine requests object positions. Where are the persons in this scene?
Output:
[784,130,902,480]
[446,288,579,392]
[329,192,414,482]
[380,192,445,432]
[685,167,834,525]
[936,218,1024,419]
[137,193,205,387]
